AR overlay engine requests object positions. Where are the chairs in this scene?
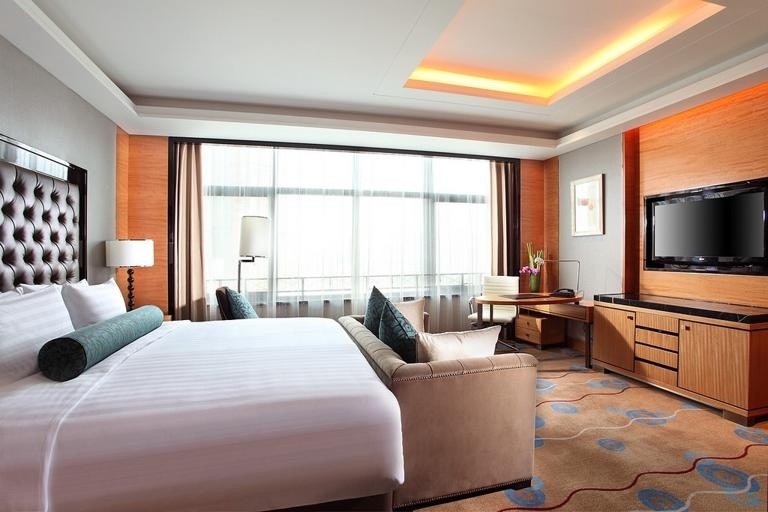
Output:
[215,286,235,320]
[468,276,519,339]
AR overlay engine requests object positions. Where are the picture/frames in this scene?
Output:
[570,174,605,237]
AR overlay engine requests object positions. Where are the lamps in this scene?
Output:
[105,239,155,312]
[237,216,268,294]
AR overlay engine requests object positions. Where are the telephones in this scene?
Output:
[550,288,575,298]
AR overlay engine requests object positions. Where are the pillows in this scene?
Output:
[378,298,420,365]
[0,283,75,387]
[362,285,387,338]
[226,288,259,318]
[391,297,426,332]
[20,279,90,295]
[37,303,165,384]
[416,325,501,362]
[61,277,128,330]
[0,287,23,300]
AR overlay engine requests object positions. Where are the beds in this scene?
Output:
[0,315,404,512]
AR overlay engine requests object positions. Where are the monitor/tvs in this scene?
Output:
[651,188,767,265]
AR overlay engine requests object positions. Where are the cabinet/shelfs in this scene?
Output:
[515,310,568,351]
[591,292,768,428]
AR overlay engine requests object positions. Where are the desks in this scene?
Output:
[514,300,594,368]
[475,292,584,330]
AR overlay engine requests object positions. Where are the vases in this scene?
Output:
[529,274,540,292]
[519,242,545,276]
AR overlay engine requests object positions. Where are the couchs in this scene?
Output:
[338,310,539,512]
